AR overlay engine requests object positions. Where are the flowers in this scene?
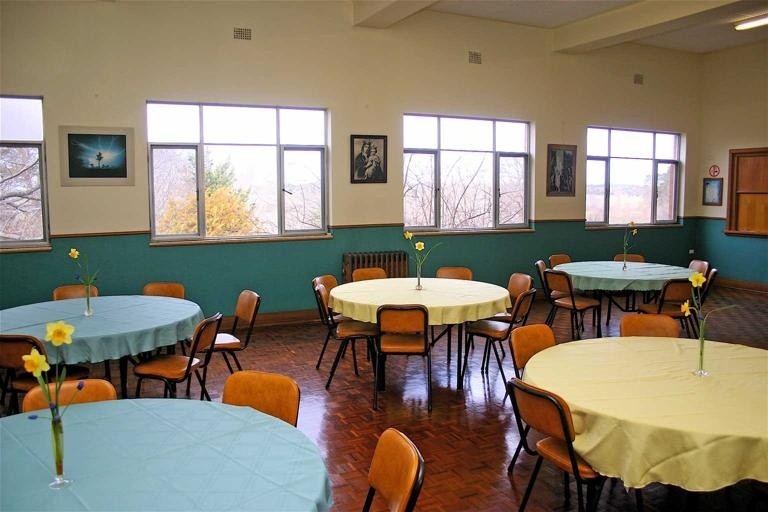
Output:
[67,247,108,286]
[23,321,75,477]
[680,272,739,370]
[400,228,442,263]
[618,220,640,249]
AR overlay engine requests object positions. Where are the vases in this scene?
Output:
[416,264,422,290]
[692,338,711,376]
[50,419,71,490]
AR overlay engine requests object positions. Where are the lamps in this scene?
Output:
[730,14,768,31]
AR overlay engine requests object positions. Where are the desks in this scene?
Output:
[521,337,767,512]
[0,398,334,512]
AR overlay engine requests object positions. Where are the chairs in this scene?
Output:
[363,428,426,512]
[0,283,260,417]
[508,325,556,511]
[222,370,300,427]
[535,254,719,340]
[312,267,537,413]
[620,314,679,337]
[507,377,642,511]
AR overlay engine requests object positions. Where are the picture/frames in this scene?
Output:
[350,135,387,183]
[702,178,723,206]
[546,144,577,197]
[58,125,136,187]
